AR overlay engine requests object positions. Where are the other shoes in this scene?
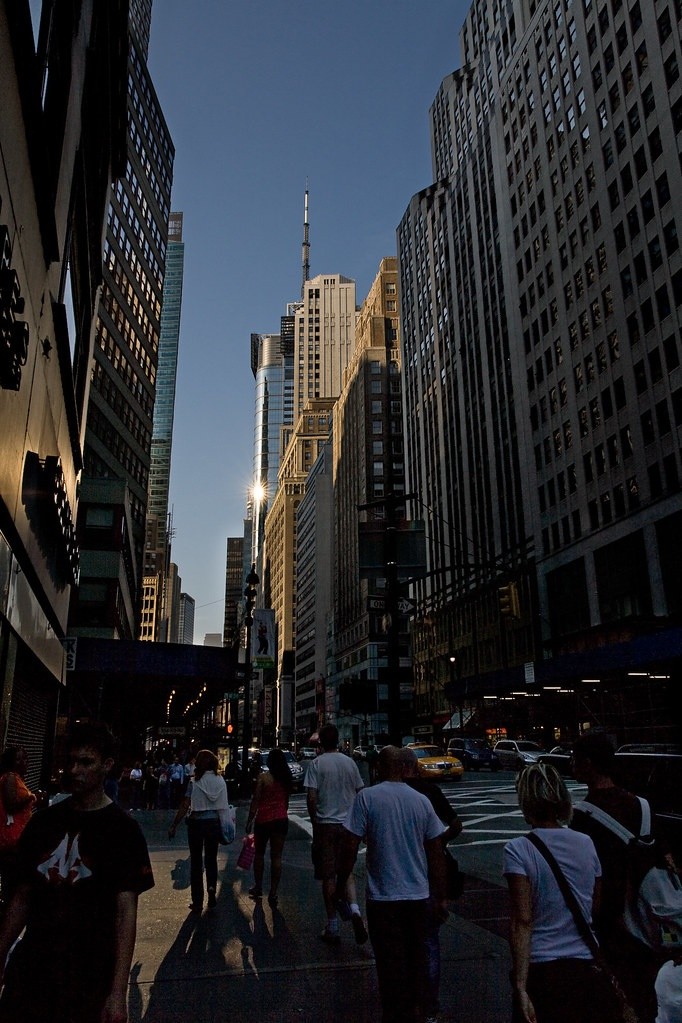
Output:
[248,887,262,896]
[207,887,216,908]
[316,925,340,941]
[352,913,367,945]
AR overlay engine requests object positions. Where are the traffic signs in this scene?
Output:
[367,595,416,617]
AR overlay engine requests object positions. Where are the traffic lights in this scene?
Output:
[225,723,236,735]
[499,584,516,618]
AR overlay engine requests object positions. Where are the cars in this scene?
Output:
[403,745,462,781]
[300,747,317,757]
[354,745,383,759]
[238,745,257,768]
[548,746,579,778]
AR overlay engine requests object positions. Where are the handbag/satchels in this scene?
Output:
[221,805,238,845]
[444,845,462,901]
[237,832,256,870]
[597,958,640,1023]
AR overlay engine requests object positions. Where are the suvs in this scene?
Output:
[249,748,304,791]
[448,738,494,770]
[492,741,547,771]
[613,743,682,869]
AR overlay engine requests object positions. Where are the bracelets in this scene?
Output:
[30,794,37,803]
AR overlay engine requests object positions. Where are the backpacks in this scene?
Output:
[570,795,682,952]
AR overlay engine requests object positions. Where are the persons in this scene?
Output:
[246,750,297,900]
[97,753,263,812]
[0,720,155,1023]
[168,750,229,911]
[568,733,682,1023]
[0,747,73,850]
[333,737,463,1023]
[303,723,369,944]
[503,758,602,1023]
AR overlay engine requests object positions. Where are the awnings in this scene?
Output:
[442,711,475,729]
